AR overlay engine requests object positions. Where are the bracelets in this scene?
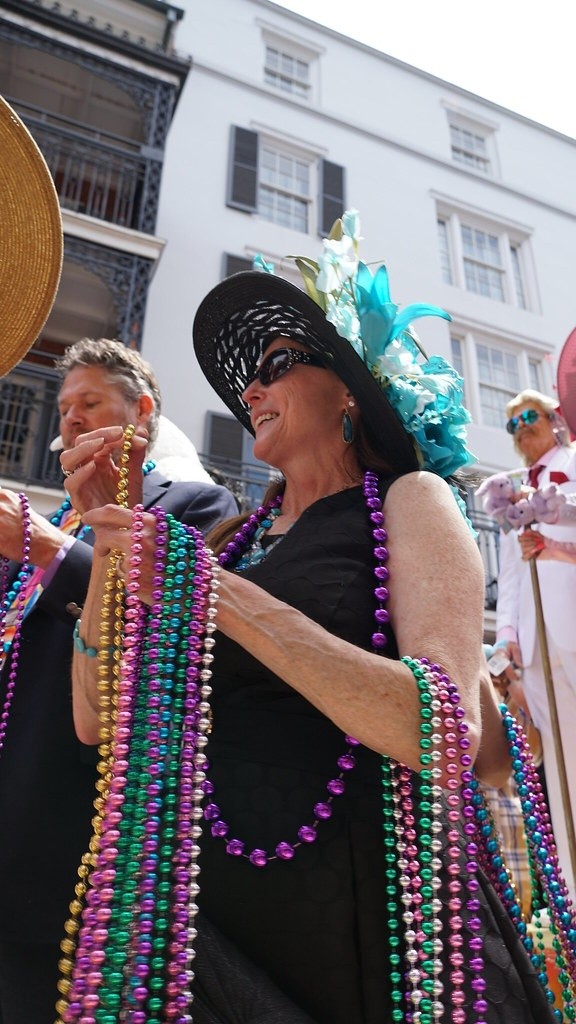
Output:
[546,510,559,524]
[71,620,120,660]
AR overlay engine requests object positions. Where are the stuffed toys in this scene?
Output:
[506,483,566,526]
[475,468,530,533]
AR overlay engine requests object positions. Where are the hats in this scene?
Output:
[192,208,479,479]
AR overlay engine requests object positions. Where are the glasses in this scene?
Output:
[506,409,550,434]
[245,347,336,387]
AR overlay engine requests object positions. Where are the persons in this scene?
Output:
[61,208,555,1024]
[51,414,215,484]
[475,391,576,911]
[0,341,240,1024]
[474,648,513,790]
[518,529,576,565]
[483,641,544,919]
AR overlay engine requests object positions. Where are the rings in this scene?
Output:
[116,557,126,577]
[62,463,81,478]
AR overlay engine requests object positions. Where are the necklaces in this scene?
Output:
[0,422,576,1024]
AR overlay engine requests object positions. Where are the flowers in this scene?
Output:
[252,211,477,477]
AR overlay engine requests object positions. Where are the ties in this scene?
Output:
[529,465,542,489]
